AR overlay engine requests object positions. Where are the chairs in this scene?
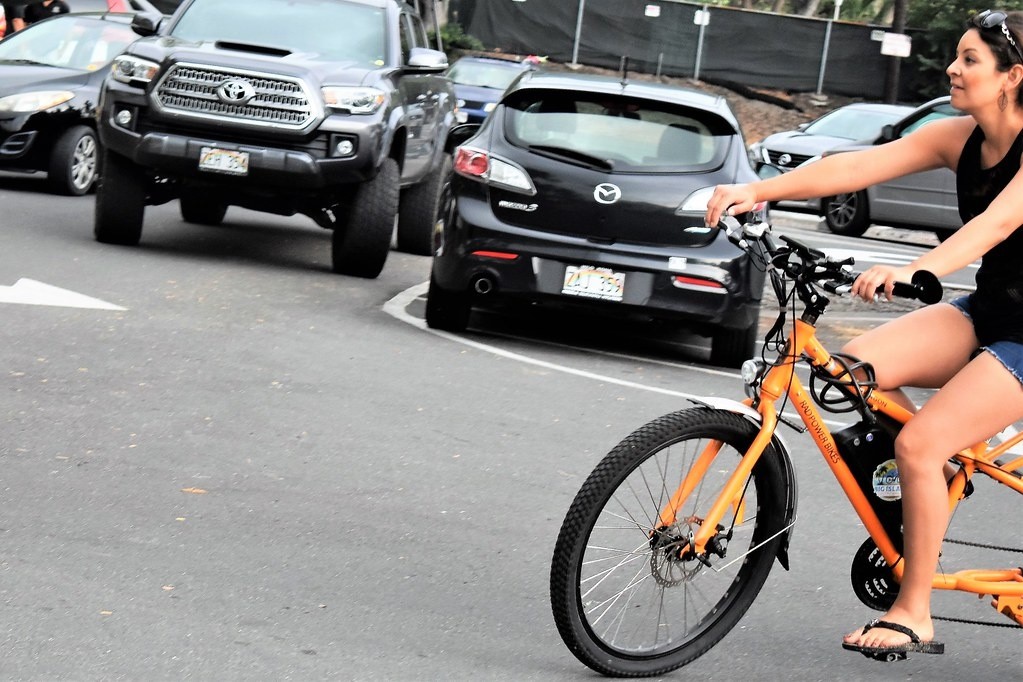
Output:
[529,99,581,152]
[643,125,702,170]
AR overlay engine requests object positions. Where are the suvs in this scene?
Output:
[94,0,459,278]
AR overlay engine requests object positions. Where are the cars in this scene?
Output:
[748,94,972,243]
[440,56,542,125]
[0,0,164,196]
[426,78,771,371]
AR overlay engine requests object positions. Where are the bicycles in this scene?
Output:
[549,203,1023,678]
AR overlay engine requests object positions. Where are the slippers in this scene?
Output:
[842,618,944,653]
[947,473,974,500]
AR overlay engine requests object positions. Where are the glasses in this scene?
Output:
[972,9,1023,64]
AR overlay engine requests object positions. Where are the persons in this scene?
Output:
[0,0,26,37]
[23,0,69,26]
[704,8,1023,656]
